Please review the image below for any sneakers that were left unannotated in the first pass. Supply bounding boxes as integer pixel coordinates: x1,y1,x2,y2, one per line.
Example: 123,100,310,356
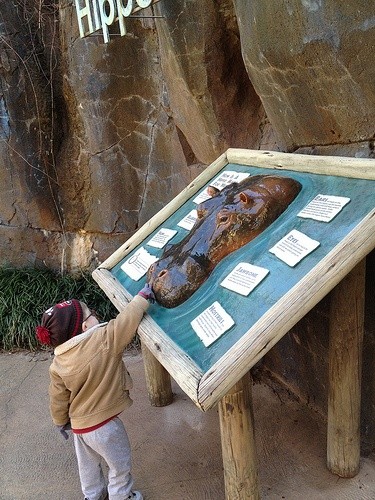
85,486,107,500
124,490,143,500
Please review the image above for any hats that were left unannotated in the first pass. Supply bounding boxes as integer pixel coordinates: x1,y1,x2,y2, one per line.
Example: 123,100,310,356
35,299,83,346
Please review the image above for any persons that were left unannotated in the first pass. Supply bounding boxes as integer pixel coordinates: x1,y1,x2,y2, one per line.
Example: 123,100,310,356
36,283,154,500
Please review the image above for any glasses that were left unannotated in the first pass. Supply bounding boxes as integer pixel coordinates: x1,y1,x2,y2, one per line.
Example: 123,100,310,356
83,309,96,321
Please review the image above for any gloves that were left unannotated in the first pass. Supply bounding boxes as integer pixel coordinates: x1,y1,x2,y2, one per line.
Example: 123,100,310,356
56,422,72,440
137,283,155,304
